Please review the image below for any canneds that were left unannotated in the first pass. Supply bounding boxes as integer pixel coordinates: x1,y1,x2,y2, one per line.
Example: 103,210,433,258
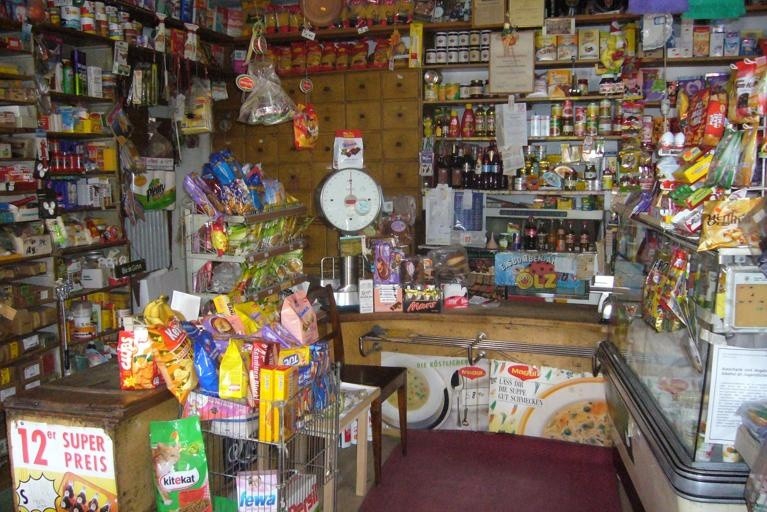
424,69,490,100
550,73,623,137
49,0,137,48
425,29,492,65
514,145,597,191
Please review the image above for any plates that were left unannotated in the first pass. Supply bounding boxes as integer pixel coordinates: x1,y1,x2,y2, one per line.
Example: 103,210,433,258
380,385,450,431
518,377,606,447
380,353,443,425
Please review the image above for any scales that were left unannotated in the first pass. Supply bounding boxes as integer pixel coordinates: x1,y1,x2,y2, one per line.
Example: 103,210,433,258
315,167,383,310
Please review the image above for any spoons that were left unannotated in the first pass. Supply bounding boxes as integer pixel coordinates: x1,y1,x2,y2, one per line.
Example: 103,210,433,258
449,369,464,428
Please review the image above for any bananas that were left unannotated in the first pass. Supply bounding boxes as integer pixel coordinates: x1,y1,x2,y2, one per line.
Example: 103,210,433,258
143,294,175,326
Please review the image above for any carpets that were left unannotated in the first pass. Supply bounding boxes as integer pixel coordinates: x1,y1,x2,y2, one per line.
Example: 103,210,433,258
359,424,625,511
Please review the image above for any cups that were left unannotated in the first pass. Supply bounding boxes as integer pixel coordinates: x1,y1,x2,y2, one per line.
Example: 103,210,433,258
514,178,527,191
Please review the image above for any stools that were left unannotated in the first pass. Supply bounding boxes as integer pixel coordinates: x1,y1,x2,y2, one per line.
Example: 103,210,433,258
253,375,381,511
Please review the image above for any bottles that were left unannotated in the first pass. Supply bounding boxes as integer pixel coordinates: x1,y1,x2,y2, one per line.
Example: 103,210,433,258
434,141,506,189
601,167,612,192
598,74,623,95
523,214,589,253
434,102,496,137
583,164,596,181
611,111,622,137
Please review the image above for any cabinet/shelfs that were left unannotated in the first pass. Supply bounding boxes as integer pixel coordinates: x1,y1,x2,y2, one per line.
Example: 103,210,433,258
213,63,421,280
2,1,236,512
422,19,641,194
591,2,766,512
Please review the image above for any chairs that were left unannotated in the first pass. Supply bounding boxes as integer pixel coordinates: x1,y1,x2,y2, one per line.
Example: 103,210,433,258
303,282,408,485
